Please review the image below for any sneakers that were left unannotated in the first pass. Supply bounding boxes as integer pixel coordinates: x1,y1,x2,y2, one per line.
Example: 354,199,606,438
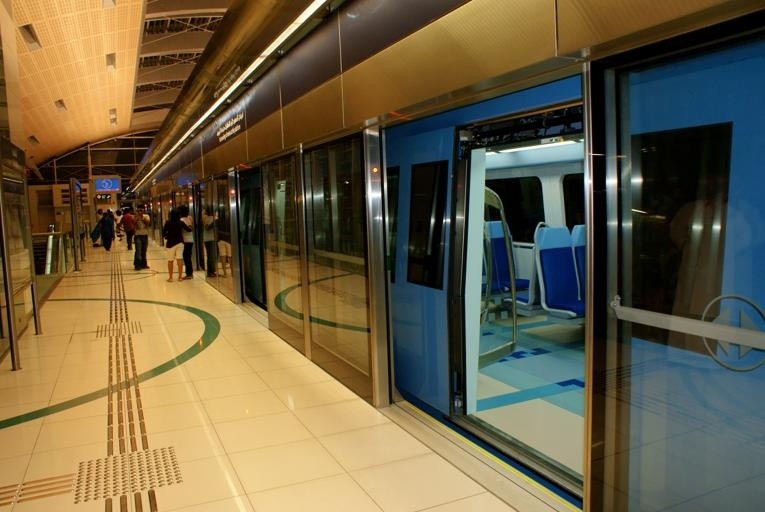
135,265,149,270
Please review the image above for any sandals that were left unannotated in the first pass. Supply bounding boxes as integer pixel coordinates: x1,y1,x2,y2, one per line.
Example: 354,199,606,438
221,274,228,277
167,276,193,282
207,272,218,277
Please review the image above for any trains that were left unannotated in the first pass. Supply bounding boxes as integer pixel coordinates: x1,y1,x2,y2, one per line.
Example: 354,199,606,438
122,0,765,512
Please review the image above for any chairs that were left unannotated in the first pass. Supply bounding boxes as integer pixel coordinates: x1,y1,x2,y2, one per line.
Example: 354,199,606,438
482,220,587,321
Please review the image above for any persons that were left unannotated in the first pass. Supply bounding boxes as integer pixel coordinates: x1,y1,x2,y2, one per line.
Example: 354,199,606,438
96,207,135,251
666,173,752,358
162,205,193,282
203,206,233,278
131,205,150,270
